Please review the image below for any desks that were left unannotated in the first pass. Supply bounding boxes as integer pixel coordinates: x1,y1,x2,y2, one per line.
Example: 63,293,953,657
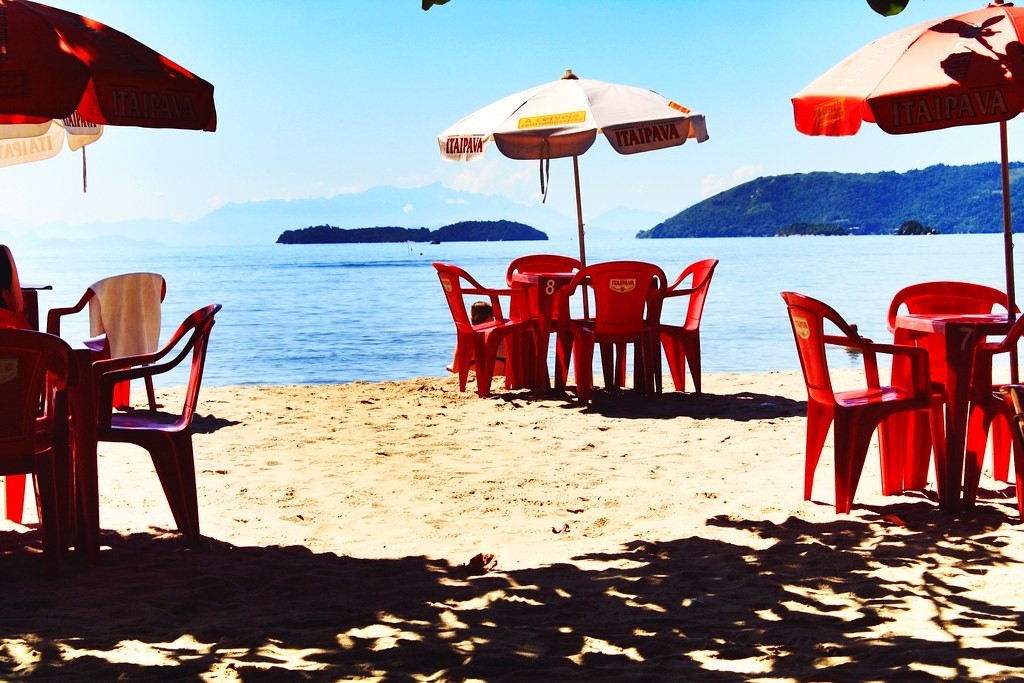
510,271,661,398
895,314,1024,516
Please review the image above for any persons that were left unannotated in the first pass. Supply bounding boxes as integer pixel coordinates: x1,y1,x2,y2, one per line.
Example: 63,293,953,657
446,301,505,376
0,244,31,330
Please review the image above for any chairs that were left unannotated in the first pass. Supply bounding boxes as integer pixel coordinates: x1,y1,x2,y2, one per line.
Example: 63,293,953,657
431,255,718,408
781,280,1023,525
0,244,222,565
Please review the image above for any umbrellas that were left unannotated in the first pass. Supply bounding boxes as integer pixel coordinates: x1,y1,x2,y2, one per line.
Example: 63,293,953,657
437,68,709,320
791,0,1024,384
0,0,217,170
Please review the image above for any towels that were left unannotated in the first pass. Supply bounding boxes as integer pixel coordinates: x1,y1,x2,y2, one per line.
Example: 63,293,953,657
87,272,163,360
